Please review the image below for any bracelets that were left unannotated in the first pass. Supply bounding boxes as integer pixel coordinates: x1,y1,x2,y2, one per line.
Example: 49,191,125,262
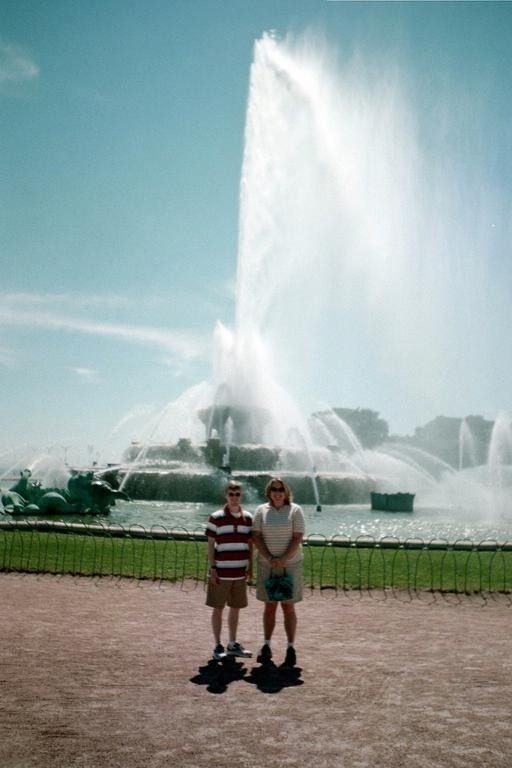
268,556,273,561
210,565,217,569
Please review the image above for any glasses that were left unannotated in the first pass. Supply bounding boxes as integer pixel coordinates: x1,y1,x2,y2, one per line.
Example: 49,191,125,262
228,492,241,496
270,486,284,491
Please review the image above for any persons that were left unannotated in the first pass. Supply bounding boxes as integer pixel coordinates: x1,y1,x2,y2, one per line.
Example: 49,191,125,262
205,481,253,661
253,478,305,664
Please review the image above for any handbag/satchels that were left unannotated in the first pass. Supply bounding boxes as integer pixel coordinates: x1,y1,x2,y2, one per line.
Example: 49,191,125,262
264,574,293,602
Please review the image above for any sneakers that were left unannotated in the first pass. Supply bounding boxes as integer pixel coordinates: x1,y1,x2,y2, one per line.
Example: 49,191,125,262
286,645,297,660
257,643,271,661
212,642,253,661
